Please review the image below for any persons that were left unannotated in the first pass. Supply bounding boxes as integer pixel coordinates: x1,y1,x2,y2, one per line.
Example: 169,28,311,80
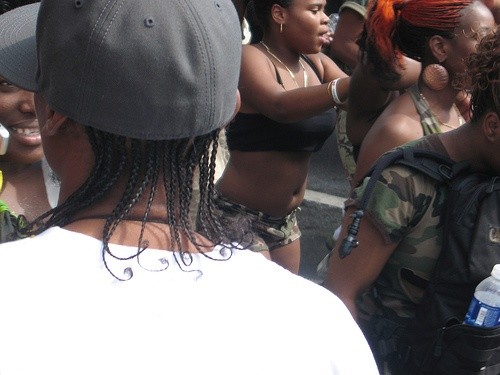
0,0,500,375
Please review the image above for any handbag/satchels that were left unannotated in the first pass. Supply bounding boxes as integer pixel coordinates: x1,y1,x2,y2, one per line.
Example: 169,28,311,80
344,61,400,145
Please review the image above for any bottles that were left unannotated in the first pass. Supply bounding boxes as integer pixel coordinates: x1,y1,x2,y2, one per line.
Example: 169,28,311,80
327,13,339,33
460,263,500,327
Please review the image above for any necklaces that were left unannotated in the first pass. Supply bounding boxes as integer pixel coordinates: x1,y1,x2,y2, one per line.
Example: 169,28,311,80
59,214,191,229
420,92,462,129
259,41,307,89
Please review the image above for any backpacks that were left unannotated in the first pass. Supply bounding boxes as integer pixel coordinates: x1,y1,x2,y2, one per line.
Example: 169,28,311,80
339,148,500,375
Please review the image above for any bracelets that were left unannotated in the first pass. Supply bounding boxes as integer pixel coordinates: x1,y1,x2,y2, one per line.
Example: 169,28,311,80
332,78,344,105
327,81,333,98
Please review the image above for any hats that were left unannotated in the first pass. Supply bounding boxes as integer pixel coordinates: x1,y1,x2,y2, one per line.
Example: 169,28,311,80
0,0,241,141
